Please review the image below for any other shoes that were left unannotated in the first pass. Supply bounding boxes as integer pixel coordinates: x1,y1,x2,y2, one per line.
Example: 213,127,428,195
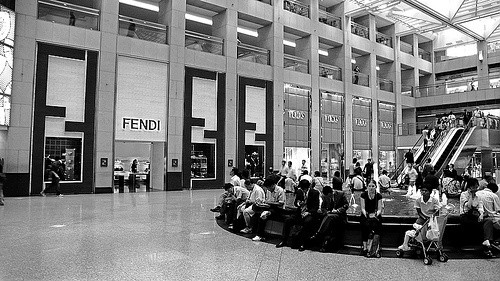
57,193,63,197
252,235,265,241
309,232,320,240
40,191,47,197
215,215,225,220
276,240,288,248
260,211,271,220
240,227,252,234
210,206,221,212
299,244,305,250
483,240,491,247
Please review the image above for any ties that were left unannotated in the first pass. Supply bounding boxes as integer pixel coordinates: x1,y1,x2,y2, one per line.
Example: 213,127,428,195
305,192,306,196
331,197,334,208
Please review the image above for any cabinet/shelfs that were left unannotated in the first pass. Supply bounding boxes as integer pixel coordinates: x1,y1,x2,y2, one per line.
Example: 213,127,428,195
190,150,207,178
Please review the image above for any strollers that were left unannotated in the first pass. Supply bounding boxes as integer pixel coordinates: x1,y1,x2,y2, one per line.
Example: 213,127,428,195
395,204,450,266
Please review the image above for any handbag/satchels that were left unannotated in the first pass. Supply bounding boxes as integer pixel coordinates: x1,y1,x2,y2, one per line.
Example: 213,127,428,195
426,215,440,241
468,209,489,219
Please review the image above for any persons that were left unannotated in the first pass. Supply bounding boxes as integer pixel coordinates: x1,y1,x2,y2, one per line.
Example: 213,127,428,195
376,33,389,46
68,12,75,26
126,18,136,37
40,158,66,198
210,148,500,258
283,0,307,16
353,66,360,85
421,106,500,152
131,159,138,173
351,21,369,39
319,12,338,27
464,75,500,92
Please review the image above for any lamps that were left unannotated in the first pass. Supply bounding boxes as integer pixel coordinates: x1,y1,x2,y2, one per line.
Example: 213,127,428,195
478,50,484,64
